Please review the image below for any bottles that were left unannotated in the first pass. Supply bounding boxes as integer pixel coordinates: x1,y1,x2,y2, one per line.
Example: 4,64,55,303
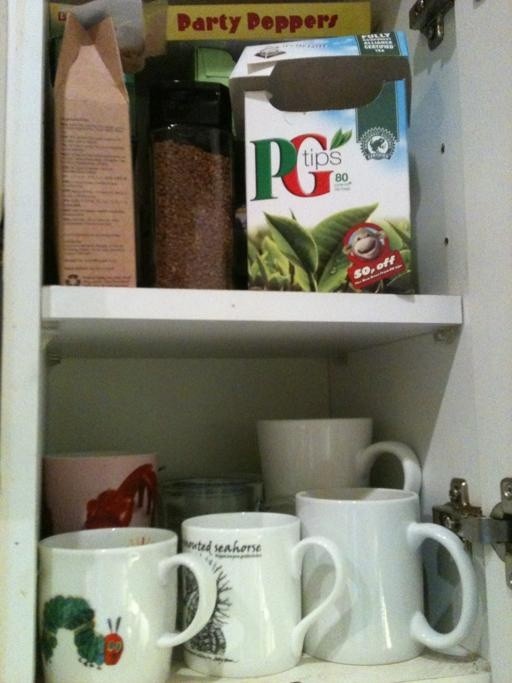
147,74,238,287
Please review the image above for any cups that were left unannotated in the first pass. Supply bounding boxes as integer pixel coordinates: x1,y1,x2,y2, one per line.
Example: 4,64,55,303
157,475,264,526
249,415,423,509
182,508,344,680
297,483,477,667
42,449,158,531
37,526,220,681
